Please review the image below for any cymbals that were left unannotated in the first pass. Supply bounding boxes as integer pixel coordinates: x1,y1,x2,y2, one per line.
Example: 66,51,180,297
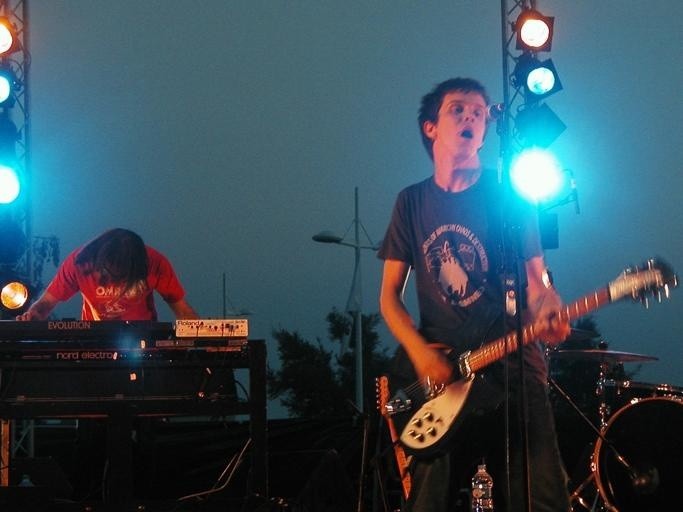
547,348,660,361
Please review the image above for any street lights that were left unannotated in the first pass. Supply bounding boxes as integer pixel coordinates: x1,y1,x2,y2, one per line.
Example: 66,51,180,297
312,187,378,407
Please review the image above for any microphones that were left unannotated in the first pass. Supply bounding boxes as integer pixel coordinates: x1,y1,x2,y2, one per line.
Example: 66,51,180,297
484,101,505,122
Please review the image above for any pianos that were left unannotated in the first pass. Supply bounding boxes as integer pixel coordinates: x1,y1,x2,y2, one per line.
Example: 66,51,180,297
0,320,251,400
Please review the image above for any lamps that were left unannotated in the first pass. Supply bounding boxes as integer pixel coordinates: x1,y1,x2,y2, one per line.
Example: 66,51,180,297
514,104,567,151
0,15,23,206
515,53,542,88
516,57,564,101
515,9,555,50
0,278,31,314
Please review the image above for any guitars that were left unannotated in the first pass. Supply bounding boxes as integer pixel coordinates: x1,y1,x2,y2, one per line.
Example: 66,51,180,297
373,257,677,464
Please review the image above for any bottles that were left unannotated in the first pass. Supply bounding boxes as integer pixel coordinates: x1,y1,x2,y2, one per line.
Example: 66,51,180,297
471,464,494,512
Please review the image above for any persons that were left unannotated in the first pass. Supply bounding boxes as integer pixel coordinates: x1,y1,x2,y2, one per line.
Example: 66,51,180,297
375,76,573,512
15,227,205,501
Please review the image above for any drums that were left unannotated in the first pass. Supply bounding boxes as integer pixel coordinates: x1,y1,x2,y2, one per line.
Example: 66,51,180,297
590,398,683,511
595,380,683,415
